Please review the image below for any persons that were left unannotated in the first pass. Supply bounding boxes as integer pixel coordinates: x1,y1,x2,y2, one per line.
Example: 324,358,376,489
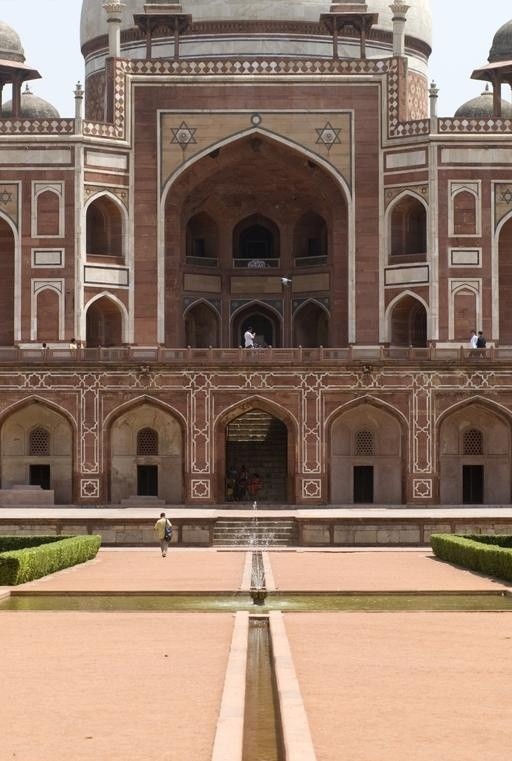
243,326,256,349
154,512,173,559
476,331,488,358
470,329,478,349
227,464,261,500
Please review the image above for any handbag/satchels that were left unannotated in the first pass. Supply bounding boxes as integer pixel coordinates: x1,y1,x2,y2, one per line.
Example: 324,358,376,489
165,518,172,541
